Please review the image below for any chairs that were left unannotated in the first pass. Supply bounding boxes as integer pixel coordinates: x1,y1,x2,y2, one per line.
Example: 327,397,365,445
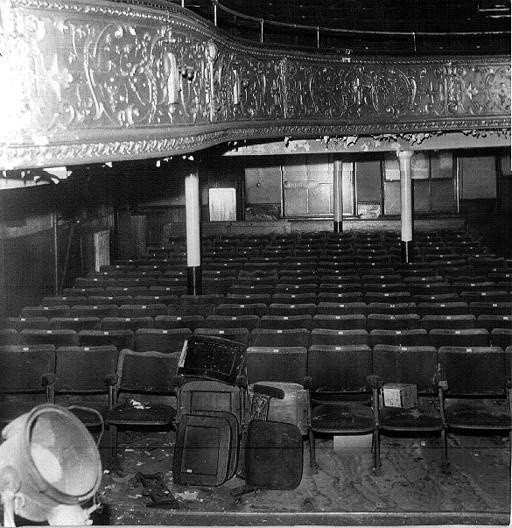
106,349,181,457
245,345,314,472
307,345,379,474
438,345,507,475
1,228,510,353
505,345,512,412
1,344,55,429
372,344,447,473
42,345,118,454
